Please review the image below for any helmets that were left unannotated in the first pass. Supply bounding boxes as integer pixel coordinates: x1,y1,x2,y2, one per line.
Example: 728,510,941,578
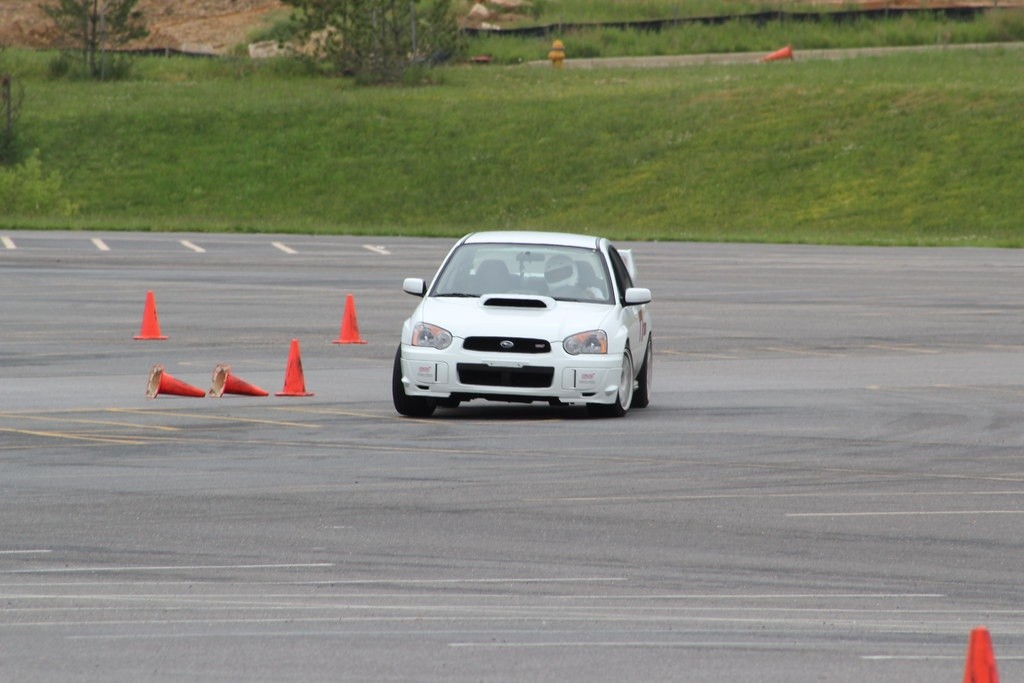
543,254,577,292
476,259,510,295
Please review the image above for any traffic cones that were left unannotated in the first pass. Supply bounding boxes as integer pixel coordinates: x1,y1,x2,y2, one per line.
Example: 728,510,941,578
132,290,169,341
145,364,207,400
760,43,794,62
274,339,315,397
207,361,269,398
330,293,370,344
962,627,999,683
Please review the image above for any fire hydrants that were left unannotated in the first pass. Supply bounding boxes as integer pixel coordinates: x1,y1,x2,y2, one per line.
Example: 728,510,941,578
548,40,565,70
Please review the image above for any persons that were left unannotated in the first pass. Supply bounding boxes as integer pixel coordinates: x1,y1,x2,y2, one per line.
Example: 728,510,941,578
544,254,605,300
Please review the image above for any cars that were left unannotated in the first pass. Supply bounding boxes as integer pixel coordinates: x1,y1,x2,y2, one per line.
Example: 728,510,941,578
391,230,653,418
